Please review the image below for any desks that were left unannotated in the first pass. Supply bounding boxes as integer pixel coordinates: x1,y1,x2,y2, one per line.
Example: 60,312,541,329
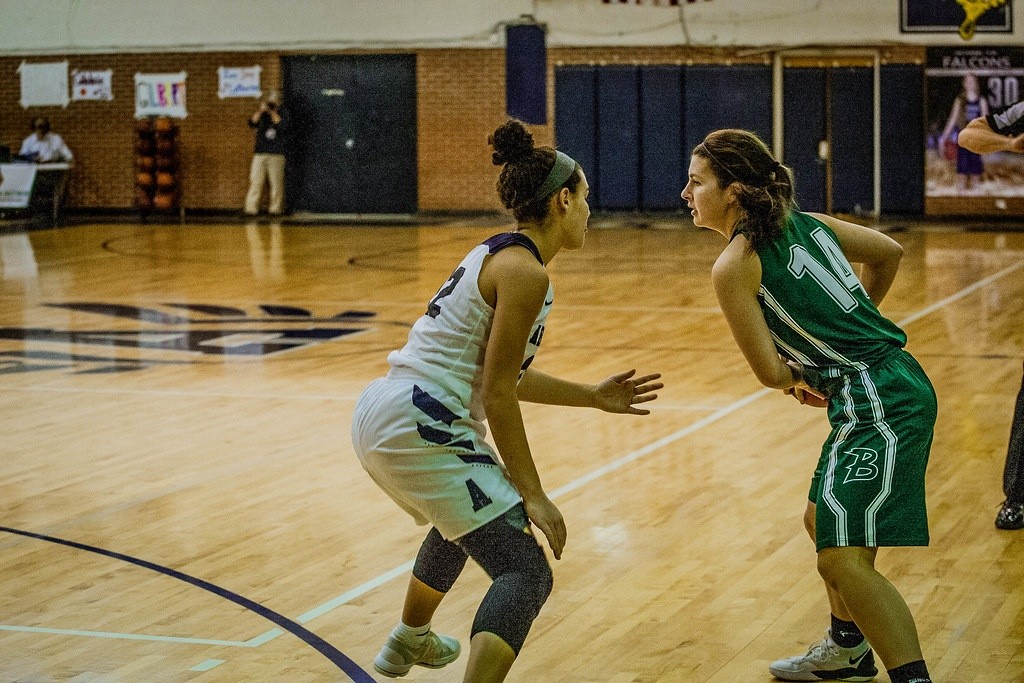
0,160,75,232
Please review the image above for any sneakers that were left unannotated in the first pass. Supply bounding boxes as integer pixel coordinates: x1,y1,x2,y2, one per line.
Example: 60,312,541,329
769,626,880,682
371,626,460,678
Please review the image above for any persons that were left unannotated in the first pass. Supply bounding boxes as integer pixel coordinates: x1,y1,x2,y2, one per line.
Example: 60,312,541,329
959,101,1024,529
239,90,288,221
350,120,665,682
683,128,937,683
19,119,77,219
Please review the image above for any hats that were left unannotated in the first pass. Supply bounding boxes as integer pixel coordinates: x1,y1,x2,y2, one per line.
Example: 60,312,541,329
267,88,287,103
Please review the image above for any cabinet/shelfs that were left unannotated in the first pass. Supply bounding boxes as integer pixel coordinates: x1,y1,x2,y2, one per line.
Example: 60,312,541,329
130,123,187,225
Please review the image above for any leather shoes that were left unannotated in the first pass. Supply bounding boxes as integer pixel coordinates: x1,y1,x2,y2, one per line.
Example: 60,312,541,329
995,495,1024,530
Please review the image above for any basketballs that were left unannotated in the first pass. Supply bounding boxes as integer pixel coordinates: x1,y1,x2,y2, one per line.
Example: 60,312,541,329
779,354,828,409
133,114,180,211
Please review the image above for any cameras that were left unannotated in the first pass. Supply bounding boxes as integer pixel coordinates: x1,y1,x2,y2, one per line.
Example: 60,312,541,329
268,101,277,109
